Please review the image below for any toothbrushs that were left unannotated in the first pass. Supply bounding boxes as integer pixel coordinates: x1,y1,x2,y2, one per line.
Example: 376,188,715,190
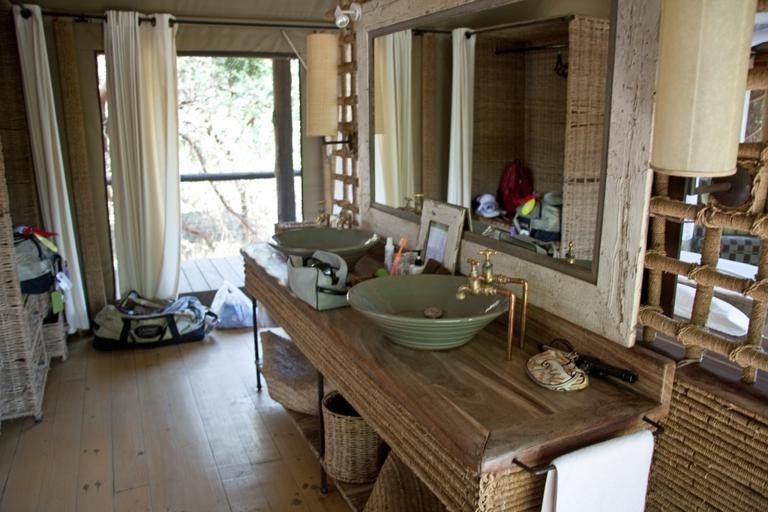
390,238,409,274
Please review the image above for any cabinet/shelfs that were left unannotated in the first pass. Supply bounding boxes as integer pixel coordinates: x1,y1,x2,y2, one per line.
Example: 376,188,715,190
239,291,675,512
0,43,67,428
418,15,612,259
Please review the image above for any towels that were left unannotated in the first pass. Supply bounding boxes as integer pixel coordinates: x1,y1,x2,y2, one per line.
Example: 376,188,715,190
541,429,656,511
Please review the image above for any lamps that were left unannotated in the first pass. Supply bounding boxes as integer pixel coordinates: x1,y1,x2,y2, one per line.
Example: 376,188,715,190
304,35,357,157
331,2,364,29
651,1,753,208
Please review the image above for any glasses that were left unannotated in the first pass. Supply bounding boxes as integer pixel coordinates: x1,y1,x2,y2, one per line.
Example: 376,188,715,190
304,257,334,277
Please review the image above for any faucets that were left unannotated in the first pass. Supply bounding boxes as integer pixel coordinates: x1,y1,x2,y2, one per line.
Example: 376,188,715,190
315,199,331,226
478,249,529,351
457,256,517,362
333,196,360,230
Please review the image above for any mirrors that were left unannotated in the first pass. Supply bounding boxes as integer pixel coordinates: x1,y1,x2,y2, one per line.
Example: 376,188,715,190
369,2,620,285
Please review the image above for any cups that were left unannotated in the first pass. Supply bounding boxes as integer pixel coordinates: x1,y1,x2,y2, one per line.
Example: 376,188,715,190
392,253,412,276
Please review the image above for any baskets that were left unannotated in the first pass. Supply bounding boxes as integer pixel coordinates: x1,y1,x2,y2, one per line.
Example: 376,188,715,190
323,388,385,488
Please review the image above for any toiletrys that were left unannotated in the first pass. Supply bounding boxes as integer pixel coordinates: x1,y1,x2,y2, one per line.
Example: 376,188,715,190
384,236,395,271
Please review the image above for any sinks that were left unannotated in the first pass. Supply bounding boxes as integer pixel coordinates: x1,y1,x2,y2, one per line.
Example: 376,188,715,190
345,272,512,352
273,224,383,272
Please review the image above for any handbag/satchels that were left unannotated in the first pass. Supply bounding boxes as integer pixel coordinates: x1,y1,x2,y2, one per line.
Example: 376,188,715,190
525,349,590,394
90,291,217,353
286,248,354,314
500,162,536,217
513,192,562,242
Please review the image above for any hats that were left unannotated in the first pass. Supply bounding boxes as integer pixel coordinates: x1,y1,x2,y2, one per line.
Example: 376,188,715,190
473,193,508,219
